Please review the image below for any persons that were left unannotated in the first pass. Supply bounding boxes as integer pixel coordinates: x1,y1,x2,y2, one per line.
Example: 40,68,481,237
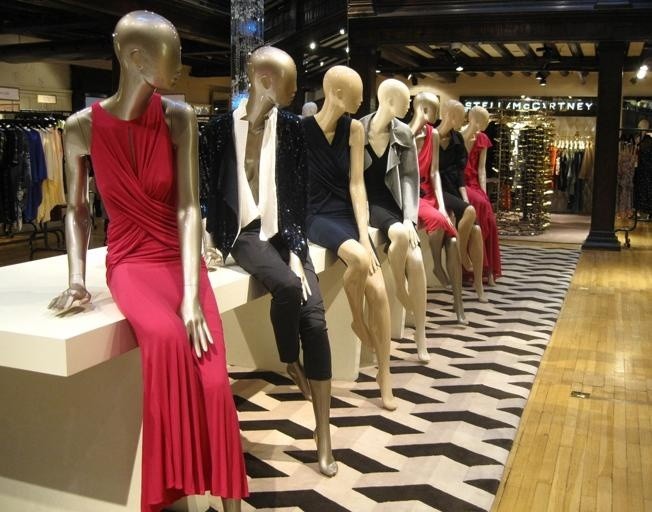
345,76,431,365
189,43,338,478
453,104,503,285
428,97,491,305
45,9,251,510
395,90,473,327
291,63,399,412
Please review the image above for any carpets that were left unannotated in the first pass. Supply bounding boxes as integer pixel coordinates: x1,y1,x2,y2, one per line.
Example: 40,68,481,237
203,241,582,512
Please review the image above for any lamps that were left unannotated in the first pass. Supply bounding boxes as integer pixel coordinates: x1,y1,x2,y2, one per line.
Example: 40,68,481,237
402,73,418,85
535,71,547,86
